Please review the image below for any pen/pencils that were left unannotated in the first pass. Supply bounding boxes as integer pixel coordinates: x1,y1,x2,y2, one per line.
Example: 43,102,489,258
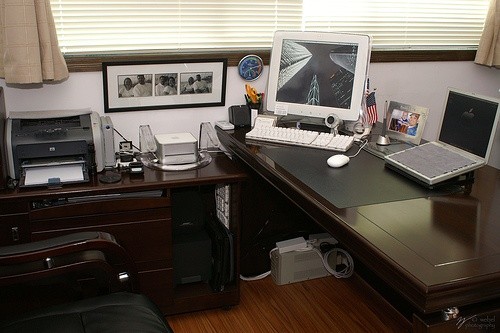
244,92,264,103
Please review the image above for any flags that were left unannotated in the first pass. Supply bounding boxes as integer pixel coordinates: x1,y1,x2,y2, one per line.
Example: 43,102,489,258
365,91,378,125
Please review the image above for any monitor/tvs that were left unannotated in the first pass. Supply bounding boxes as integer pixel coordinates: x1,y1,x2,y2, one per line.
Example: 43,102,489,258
266,31,371,122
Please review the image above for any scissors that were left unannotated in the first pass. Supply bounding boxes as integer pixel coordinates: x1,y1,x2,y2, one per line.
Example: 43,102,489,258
245,84,257,103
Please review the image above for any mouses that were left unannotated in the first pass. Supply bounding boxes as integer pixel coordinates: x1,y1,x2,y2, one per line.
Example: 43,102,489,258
326,154,350,167
377,134,390,145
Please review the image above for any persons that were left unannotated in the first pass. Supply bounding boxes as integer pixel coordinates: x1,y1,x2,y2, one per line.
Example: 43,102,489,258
134,75,152,97
155,76,167,95
193,74,209,94
181,77,194,94
119,78,134,98
161,77,177,95
393,111,421,136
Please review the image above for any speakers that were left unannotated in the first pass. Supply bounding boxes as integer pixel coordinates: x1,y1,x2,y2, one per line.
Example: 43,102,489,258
172,191,207,235
228,105,250,125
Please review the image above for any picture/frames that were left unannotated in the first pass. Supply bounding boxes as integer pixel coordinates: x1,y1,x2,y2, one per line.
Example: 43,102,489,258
381,101,429,144
102,58,227,113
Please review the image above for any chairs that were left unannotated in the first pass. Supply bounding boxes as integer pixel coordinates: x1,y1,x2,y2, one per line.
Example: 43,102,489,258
0,232,173,333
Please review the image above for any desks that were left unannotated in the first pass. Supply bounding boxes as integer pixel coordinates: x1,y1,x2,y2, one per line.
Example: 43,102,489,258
217,122,500,333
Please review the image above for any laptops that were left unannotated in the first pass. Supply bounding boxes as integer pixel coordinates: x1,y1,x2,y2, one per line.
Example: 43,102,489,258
383,86,500,185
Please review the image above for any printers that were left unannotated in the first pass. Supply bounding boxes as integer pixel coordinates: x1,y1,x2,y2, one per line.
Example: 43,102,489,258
4,109,115,188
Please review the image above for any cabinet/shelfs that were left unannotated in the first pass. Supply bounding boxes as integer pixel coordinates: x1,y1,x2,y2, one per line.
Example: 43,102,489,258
0,148,246,317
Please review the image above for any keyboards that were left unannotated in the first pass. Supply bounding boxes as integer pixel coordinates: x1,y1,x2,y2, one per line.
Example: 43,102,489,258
245,125,354,152
